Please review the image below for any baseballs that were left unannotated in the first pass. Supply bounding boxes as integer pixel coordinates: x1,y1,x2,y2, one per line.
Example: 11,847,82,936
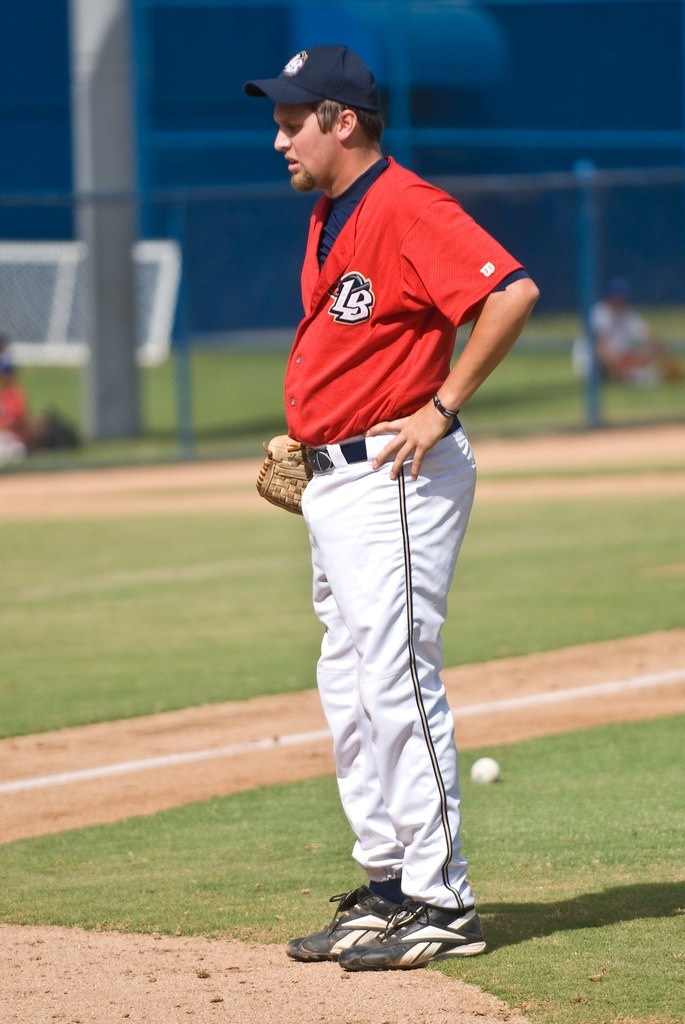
472,757,503,788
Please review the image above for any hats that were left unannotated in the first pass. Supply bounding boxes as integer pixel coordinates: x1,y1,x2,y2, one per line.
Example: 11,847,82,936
243,44,380,111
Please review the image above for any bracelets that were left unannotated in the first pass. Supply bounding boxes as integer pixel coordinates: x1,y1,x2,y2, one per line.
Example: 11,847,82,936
433,393,460,418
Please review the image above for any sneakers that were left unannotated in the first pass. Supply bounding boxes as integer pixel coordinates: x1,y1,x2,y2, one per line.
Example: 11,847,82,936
338,900,486,971
286,886,401,962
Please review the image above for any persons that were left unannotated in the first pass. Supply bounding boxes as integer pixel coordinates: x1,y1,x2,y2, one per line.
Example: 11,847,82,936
0,343,87,467
572,274,685,383
244,47,541,972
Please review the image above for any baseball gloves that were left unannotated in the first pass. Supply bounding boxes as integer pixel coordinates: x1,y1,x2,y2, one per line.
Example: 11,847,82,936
255,434,314,516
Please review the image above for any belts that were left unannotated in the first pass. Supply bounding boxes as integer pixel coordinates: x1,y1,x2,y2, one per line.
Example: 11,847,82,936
306,415,462,472
